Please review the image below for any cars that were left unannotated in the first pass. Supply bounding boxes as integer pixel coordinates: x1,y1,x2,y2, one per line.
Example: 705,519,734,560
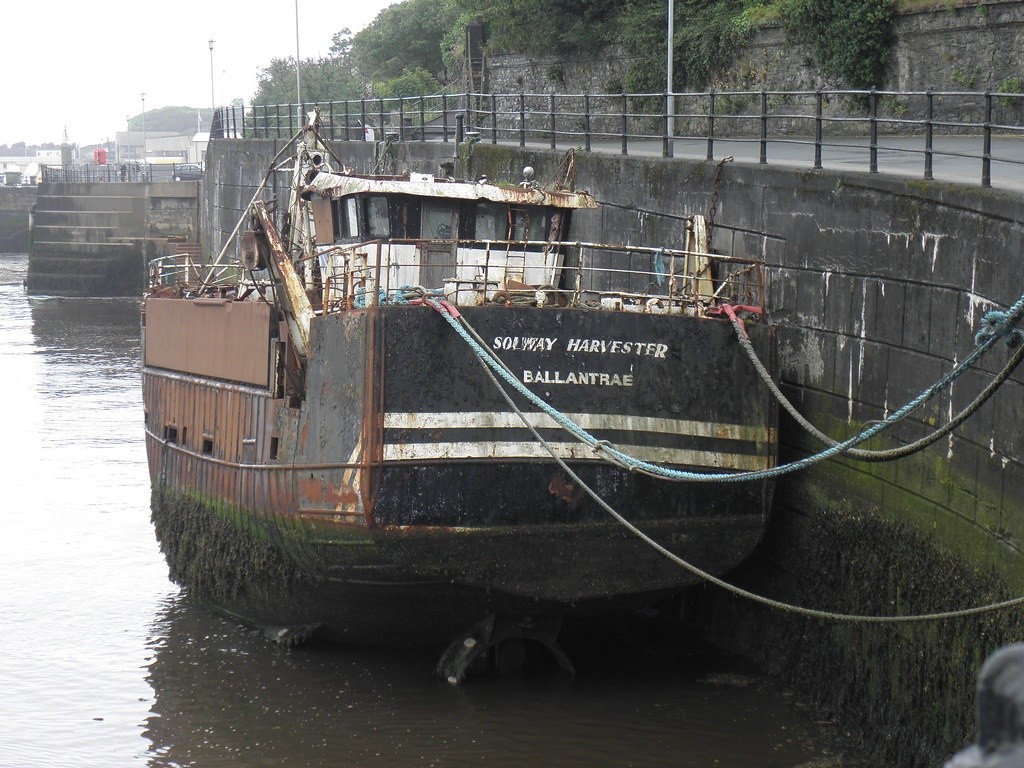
172,163,205,181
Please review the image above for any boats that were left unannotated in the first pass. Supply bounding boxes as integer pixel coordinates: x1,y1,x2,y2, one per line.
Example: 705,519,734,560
140,103,779,686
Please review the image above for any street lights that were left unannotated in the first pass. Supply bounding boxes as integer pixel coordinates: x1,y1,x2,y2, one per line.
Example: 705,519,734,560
209,37,216,113
